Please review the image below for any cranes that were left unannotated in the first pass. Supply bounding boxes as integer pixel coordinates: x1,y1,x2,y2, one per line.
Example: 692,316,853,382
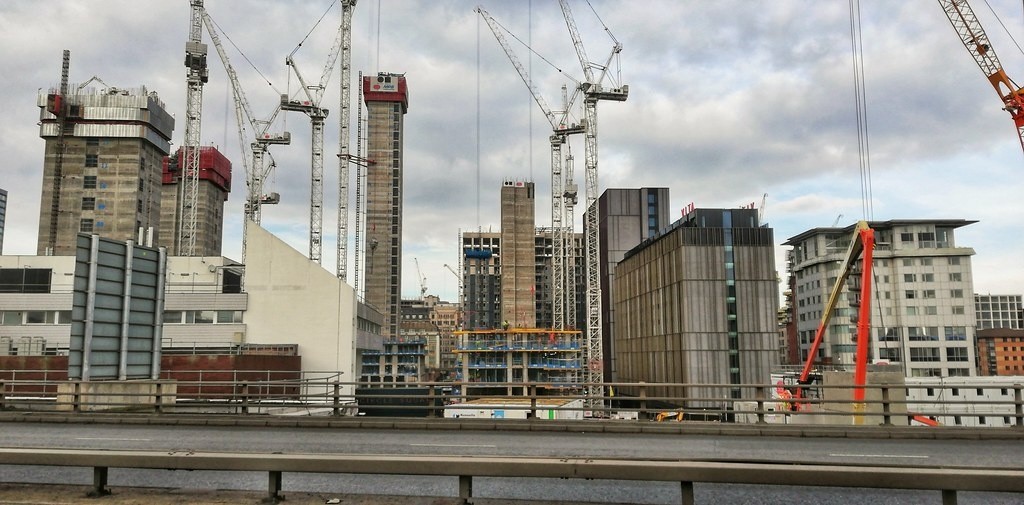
468,0,630,408
171,0,351,285
